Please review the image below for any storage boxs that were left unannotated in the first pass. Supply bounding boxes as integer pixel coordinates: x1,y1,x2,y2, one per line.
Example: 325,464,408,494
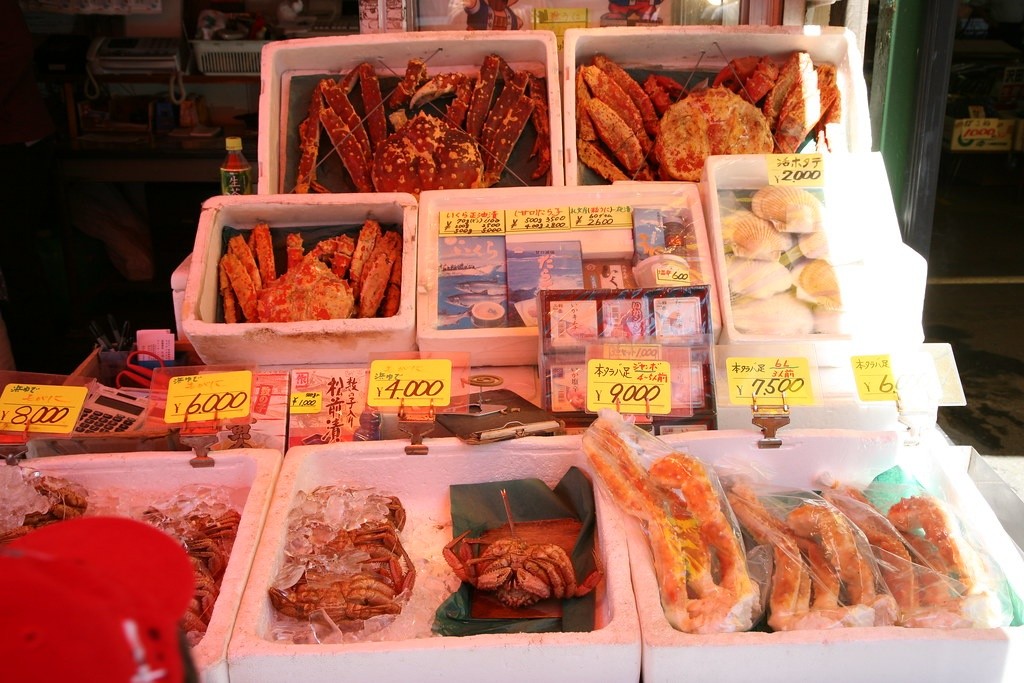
625,428,1024,683
181,191,417,362
0,447,283,683
124,0,183,39
228,434,642,683
699,150,928,345
418,183,723,366
562,23,873,183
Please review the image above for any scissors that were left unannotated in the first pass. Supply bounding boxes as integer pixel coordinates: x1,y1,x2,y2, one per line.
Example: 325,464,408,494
116,351,172,391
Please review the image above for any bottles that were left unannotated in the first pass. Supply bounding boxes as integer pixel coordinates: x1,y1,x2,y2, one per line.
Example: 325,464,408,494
219,136,252,196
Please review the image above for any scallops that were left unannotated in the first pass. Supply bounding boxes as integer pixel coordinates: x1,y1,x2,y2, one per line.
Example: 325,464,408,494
720,185,843,334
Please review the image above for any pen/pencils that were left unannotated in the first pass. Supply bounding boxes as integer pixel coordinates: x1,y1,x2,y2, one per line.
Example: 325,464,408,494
91,314,129,352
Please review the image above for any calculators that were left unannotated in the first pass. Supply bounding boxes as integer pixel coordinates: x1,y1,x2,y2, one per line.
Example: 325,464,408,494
72,382,158,433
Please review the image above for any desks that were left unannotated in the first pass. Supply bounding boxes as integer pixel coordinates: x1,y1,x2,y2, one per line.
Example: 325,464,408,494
36,141,257,333
258,29,564,193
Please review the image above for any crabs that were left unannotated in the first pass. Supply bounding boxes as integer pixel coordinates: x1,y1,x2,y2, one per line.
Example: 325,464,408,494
442,529,605,610
582,416,973,634
141,485,416,649
218,52,841,324
